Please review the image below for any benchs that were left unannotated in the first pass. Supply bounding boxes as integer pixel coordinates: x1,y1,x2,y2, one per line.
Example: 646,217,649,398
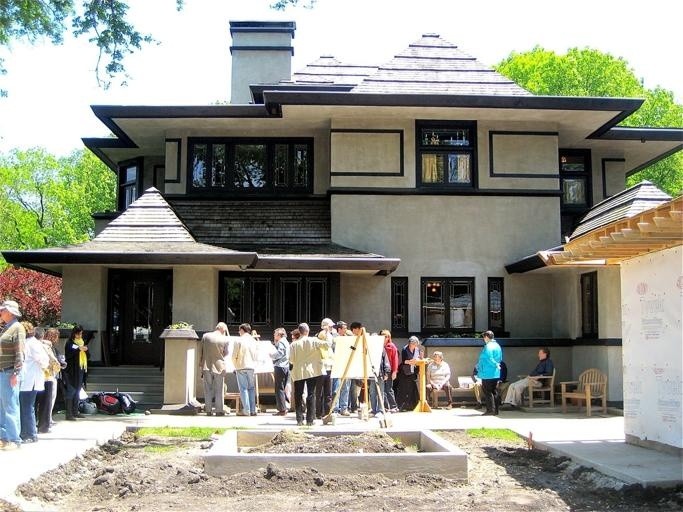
428,383,499,408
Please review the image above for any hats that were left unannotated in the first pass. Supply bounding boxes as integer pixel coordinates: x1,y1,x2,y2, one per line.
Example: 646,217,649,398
0,301,22,317
320,318,336,329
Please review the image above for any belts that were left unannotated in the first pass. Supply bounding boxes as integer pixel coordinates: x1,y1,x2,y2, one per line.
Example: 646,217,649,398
0,366,14,372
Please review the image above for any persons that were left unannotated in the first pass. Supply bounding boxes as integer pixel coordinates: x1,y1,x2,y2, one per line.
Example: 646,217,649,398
500,347,554,409
198,317,453,426
15,321,49,442
45,327,62,424
0,300,26,450
33,326,60,434
477,330,502,416
471,360,511,410
59,326,91,421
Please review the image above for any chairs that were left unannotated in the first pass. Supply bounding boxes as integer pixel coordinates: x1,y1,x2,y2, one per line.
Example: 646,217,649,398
559,367,608,416
516,367,556,408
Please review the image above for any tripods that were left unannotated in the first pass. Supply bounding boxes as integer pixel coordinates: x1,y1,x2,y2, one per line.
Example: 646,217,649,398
322,328,394,428
